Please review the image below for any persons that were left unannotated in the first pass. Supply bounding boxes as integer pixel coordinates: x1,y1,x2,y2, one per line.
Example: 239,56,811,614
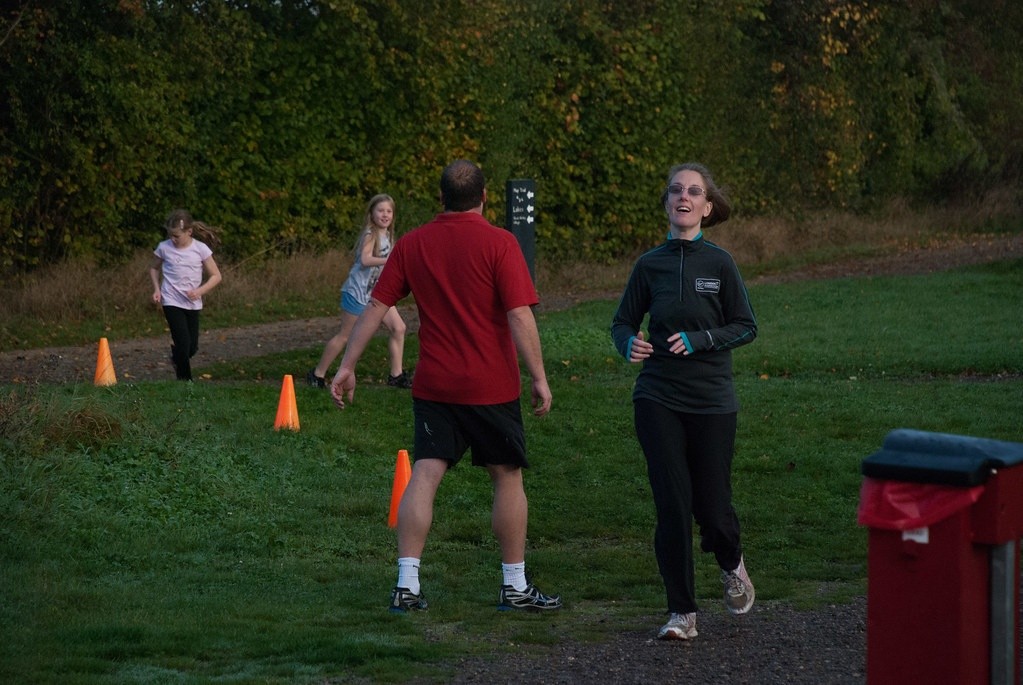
330,161,572,609
149,210,226,381
611,161,763,640
307,192,416,389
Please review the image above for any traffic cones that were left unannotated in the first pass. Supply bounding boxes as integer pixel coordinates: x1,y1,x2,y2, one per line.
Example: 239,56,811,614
273,373,300,431
93,337,118,388
385,449,413,528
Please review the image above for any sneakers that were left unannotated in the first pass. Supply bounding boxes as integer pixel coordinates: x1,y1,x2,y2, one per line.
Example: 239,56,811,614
389,587,429,613
306,369,325,388
497,581,564,611
720,553,756,614
657,612,698,639
386,370,413,389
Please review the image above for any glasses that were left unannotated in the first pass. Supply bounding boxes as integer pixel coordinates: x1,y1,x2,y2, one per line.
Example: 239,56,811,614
668,185,707,197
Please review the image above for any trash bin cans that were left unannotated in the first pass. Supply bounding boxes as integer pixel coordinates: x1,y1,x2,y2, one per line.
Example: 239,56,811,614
854,427,1021,684
